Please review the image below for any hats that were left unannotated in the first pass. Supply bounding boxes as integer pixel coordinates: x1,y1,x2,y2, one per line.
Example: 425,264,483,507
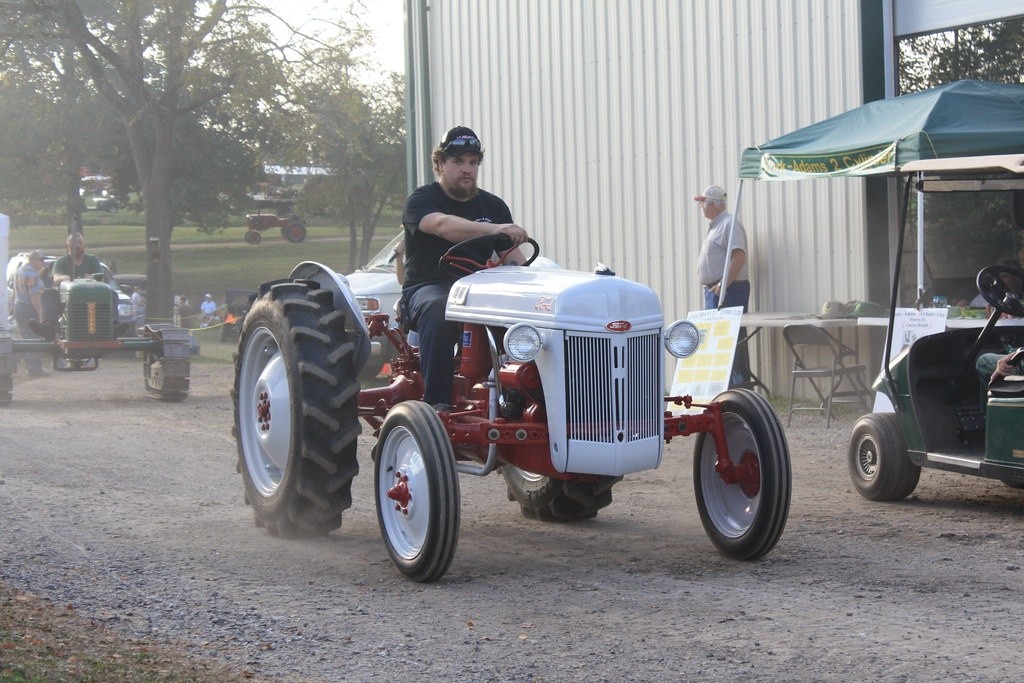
28,250,49,261
439,126,482,158
815,300,846,319
848,302,882,316
694,185,727,202
206,293,212,298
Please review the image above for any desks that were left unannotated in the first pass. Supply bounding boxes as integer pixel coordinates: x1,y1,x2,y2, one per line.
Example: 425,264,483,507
739,312,1024,418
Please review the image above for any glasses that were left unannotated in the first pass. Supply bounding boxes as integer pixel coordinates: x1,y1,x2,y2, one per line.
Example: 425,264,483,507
444,138,481,150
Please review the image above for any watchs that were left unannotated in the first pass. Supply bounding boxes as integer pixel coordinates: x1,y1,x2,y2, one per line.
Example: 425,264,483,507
718,283,722,287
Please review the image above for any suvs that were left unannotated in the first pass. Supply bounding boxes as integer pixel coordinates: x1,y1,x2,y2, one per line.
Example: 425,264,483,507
6,252,134,340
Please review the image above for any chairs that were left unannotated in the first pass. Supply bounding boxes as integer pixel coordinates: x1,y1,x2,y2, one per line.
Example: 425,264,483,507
989,352,1024,404
388,240,420,334
782,324,875,428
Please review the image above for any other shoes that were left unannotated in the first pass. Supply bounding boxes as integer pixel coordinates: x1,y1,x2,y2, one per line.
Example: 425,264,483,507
29,318,54,342
113,320,129,337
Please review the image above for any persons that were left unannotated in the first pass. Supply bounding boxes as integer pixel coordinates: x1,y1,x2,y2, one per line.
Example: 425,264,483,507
131,285,144,326
693,185,753,389
995,257,1024,300
29,233,105,341
199,293,216,328
399,125,528,413
976,349,1024,420
178,295,192,327
7,249,50,376
109,259,116,275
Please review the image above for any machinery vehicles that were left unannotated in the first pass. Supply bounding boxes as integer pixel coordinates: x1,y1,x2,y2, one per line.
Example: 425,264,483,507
233,235,793,587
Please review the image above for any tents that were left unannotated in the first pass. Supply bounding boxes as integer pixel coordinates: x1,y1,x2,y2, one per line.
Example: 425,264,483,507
717,78,1024,308
